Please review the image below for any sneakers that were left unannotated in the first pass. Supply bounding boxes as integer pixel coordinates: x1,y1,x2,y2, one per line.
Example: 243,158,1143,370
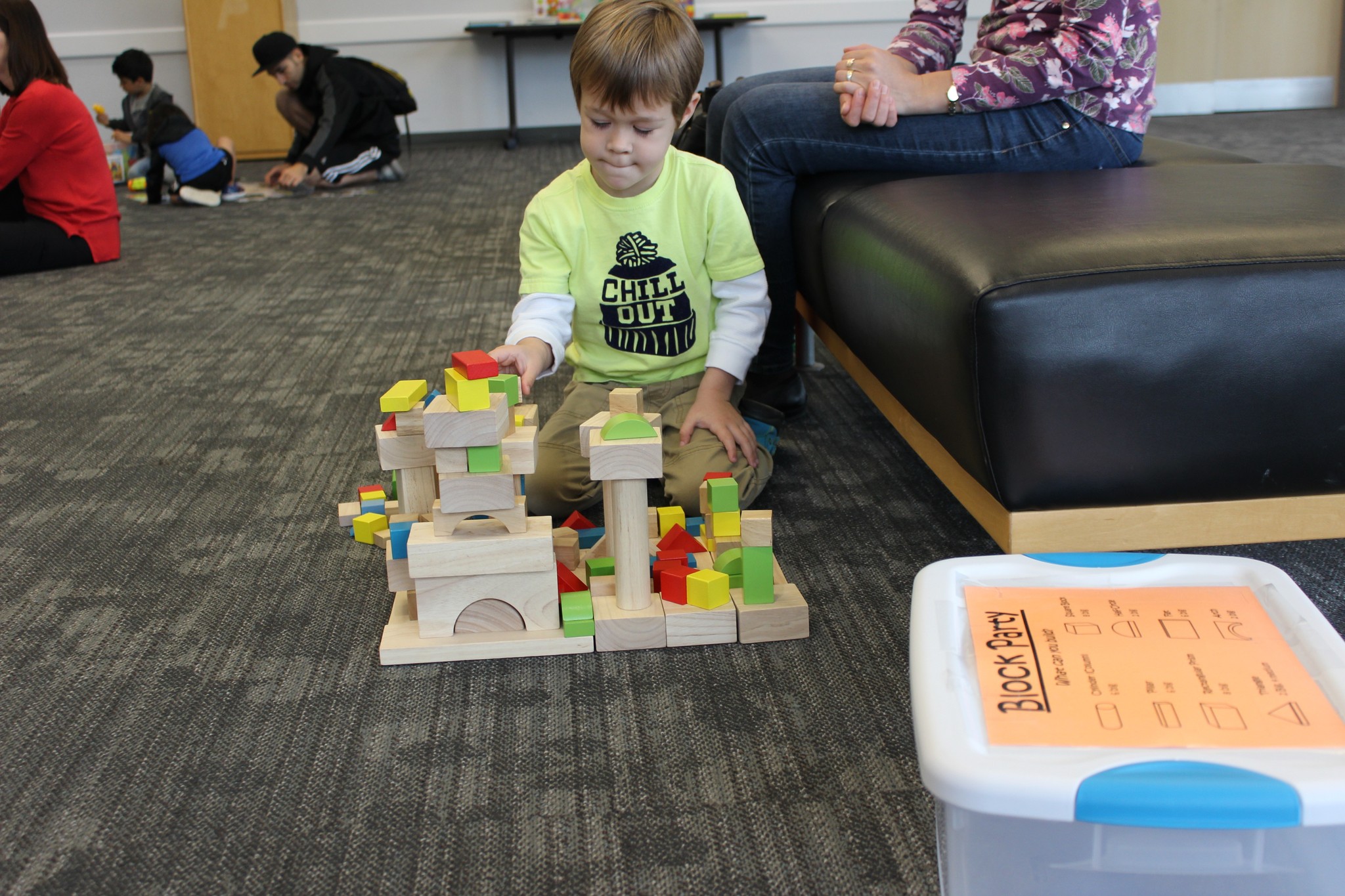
222,181,247,203
741,399,784,456
377,159,404,181
127,176,147,191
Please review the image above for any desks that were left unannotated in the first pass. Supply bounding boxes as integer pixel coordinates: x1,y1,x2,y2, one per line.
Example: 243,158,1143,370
464,12,766,153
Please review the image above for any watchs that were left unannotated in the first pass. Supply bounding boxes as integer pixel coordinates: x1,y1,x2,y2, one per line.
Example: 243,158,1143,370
946,81,959,116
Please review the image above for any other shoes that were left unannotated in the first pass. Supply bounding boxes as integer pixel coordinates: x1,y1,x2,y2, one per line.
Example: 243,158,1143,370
741,371,809,425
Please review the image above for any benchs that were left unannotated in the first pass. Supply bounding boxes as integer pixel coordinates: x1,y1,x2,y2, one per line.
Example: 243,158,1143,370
788,130,1344,557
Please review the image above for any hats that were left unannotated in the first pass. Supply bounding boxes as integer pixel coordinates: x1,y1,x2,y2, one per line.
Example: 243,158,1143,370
252,33,297,77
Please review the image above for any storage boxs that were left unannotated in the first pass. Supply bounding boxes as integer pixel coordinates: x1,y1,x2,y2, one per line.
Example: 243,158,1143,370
906,548,1345,896
103,140,140,186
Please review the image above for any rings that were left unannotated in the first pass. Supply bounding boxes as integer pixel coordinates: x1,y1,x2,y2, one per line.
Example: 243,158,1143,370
846,58,855,70
847,70,854,82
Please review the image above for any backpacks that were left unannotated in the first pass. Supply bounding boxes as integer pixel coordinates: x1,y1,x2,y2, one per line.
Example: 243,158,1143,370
330,56,417,115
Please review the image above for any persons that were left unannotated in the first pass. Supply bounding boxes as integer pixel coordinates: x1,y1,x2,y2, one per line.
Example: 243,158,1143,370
0,0,122,277
250,30,418,191
97,48,177,181
134,100,247,207
704,0,1159,413
486,1,784,531
